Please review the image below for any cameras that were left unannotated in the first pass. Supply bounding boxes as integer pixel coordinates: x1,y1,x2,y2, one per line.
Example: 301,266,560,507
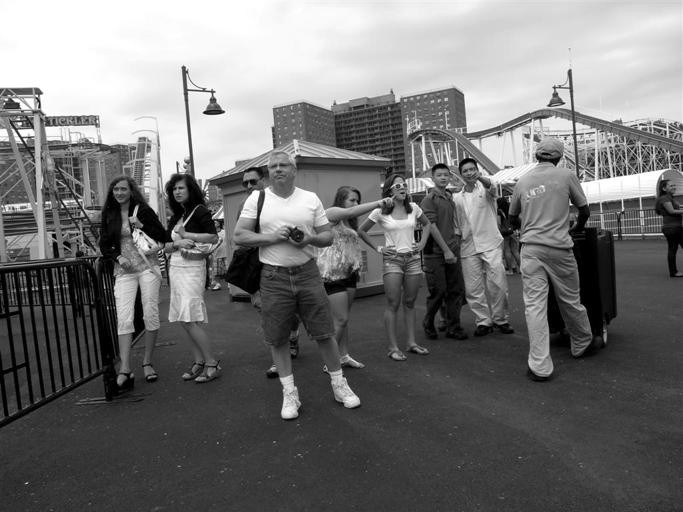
287,226,306,242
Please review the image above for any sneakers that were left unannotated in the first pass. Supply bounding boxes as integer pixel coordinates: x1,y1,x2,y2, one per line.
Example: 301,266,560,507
423,321,438,338
341,353,366,369
496,322,513,334
442,327,466,339
671,272,682,277
267,364,279,378
281,388,301,419
506,270,513,275
289,337,299,359
527,366,555,382
474,324,493,336
517,269,522,274
574,336,604,358
213,283,221,291
331,376,360,408
323,365,329,374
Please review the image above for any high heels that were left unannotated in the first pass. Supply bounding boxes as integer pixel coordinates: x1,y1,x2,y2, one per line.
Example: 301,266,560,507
112,372,134,393
142,365,158,383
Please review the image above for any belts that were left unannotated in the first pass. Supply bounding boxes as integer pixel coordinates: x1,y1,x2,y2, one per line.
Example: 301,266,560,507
395,251,418,258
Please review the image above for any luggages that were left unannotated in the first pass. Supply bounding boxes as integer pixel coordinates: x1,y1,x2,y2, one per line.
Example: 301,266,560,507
547,226,617,346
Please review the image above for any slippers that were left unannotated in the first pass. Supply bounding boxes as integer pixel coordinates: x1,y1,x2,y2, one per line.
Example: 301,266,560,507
388,348,406,360
409,344,429,355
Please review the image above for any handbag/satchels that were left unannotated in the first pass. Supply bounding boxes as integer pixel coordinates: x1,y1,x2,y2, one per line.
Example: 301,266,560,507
499,221,513,238
132,229,164,257
179,236,223,260
223,246,263,295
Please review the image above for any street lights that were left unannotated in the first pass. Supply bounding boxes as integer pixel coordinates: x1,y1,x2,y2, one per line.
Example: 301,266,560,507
547,66,583,183
179,66,225,178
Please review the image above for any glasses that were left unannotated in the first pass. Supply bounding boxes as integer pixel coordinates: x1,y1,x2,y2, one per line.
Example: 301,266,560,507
267,161,293,169
243,177,262,187
391,183,407,189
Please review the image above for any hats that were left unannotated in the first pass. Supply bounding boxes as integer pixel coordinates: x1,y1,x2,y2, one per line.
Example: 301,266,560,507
536,138,564,158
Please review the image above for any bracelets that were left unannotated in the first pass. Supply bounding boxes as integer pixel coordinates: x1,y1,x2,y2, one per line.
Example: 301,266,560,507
377,245,383,255
378,199,382,207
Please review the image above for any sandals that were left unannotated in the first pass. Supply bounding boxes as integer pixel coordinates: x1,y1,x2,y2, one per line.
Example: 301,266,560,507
181,361,204,380
195,360,222,383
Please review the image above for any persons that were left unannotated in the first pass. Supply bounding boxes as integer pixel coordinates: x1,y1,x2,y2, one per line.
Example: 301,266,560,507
235,150,362,421
156,217,225,291
99,176,166,387
319,185,396,376
236,166,300,377
70,252,94,294
509,136,602,381
164,174,222,384
421,137,521,341
358,175,432,362
655,180,683,278
